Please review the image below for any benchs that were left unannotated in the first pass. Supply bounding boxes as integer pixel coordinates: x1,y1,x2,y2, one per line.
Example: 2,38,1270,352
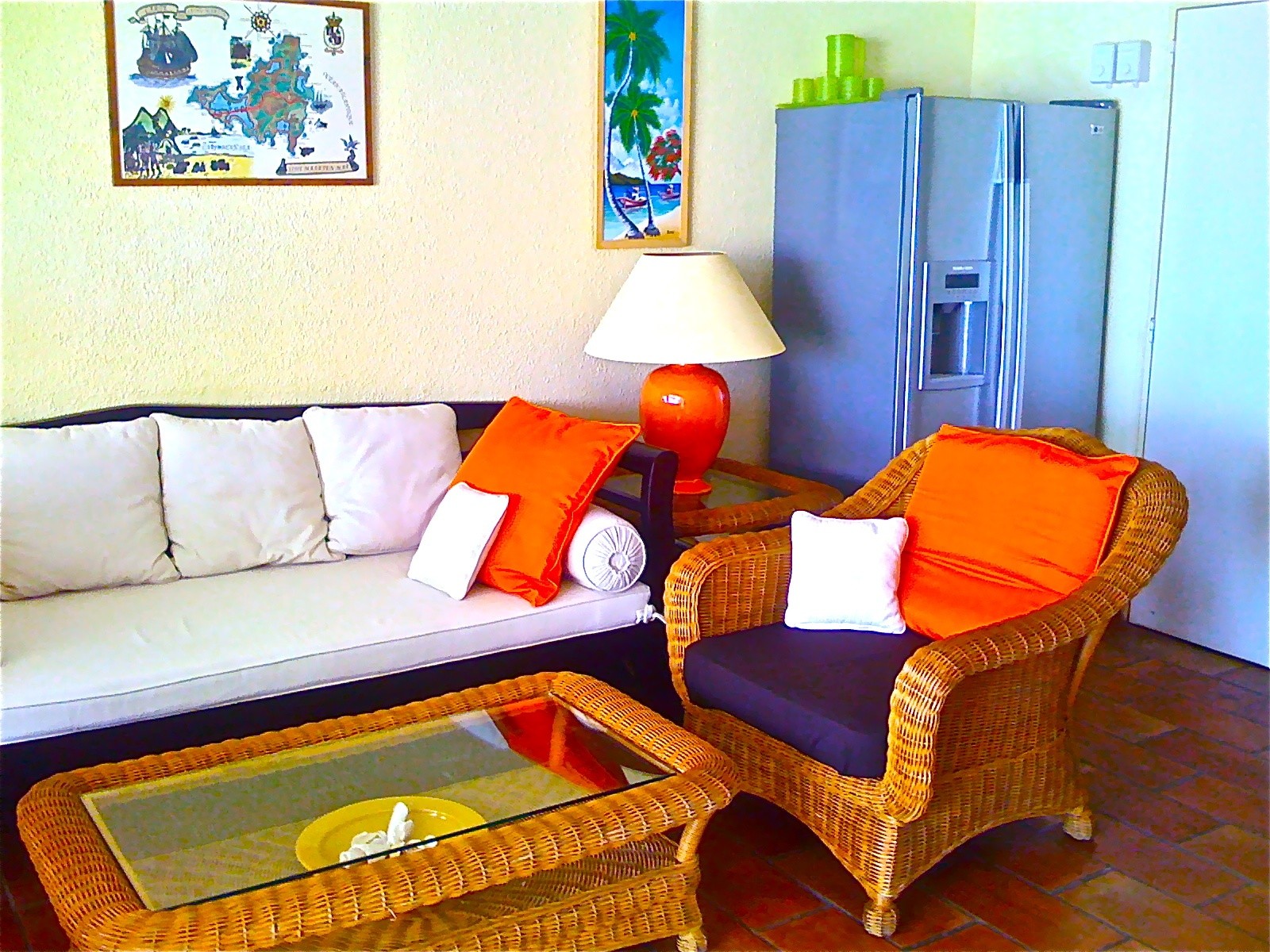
0,401,680,813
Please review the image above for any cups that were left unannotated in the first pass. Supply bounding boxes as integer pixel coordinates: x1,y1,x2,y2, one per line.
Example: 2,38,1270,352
862,77,884,102
816,76,838,103
839,76,863,102
792,78,816,104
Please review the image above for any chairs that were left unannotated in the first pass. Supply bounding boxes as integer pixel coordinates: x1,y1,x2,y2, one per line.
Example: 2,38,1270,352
662,427,1190,939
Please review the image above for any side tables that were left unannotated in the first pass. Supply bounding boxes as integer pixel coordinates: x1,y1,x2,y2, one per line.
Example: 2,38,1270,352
593,458,845,542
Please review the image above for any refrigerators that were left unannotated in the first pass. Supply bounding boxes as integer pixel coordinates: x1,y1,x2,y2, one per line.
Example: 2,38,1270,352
770,88,1118,497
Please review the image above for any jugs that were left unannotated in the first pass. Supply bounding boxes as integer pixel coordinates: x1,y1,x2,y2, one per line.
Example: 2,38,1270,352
826,34,866,101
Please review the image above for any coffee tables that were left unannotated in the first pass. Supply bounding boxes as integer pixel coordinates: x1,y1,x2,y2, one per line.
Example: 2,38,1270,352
18,670,743,952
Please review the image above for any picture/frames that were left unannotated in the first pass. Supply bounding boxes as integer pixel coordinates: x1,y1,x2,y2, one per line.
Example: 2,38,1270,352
103,0,375,188
594,0,693,249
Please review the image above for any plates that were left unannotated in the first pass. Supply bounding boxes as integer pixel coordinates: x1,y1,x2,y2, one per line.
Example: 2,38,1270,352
296,796,488,877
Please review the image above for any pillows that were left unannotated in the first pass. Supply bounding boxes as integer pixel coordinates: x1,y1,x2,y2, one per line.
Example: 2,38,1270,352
149,412,346,578
785,508,907,635
0,418,182,602
897,423,1139,642
448,394,643,609
407,481,511,600
562,504,647,594
303,402,464,556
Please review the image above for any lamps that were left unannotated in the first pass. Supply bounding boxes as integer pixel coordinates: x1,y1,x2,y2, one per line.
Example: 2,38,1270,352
584,248,785,498
